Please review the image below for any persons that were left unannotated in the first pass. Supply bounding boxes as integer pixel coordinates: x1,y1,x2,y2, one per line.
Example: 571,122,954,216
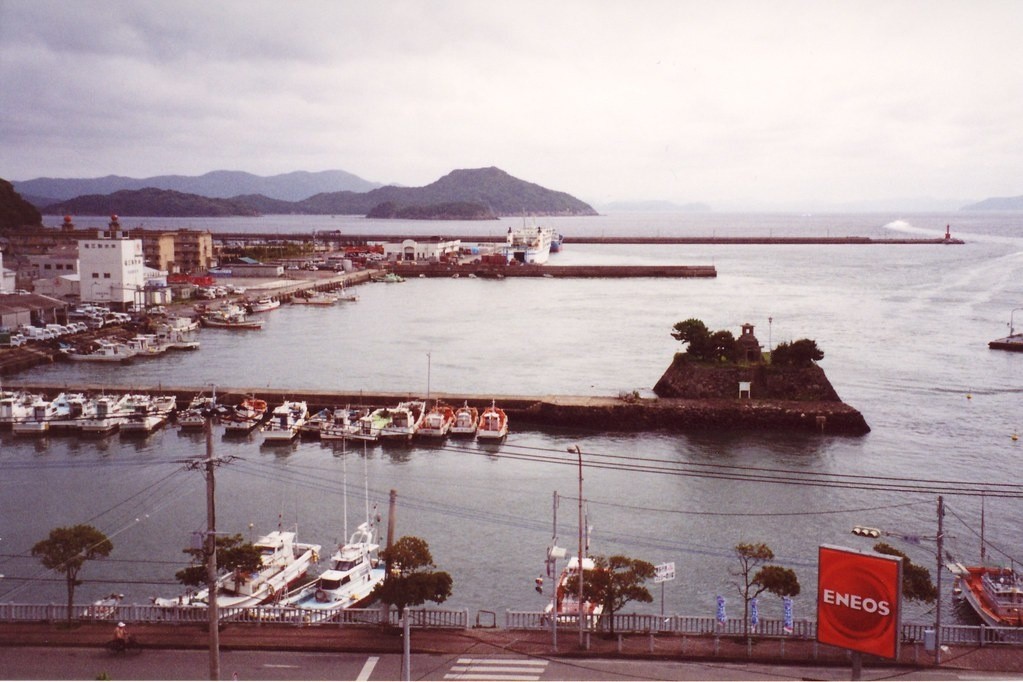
114,621,129,659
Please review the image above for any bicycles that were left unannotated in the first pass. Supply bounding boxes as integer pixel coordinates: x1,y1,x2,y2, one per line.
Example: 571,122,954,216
104,633,142,658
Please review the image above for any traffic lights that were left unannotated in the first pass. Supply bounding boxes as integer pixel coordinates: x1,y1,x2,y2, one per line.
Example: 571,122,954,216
943,562,971,580
951,587,963,604
534,577,544,594
852,525,882,539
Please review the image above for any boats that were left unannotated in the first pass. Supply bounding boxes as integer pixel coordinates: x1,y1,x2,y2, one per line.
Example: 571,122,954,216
494,205,551,265
246,433,404,627
370,270,405,282
0,282,281,363
544,526,606,629
148,485,323,624
76,592,126,620
549,227,565,253
290,277,337,305
326,281,360,302
960,491,1023,642
0,382,510,445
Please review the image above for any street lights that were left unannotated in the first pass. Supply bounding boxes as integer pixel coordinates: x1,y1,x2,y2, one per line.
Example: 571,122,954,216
425,351,432,410
566,443,585,648
767,315,773,353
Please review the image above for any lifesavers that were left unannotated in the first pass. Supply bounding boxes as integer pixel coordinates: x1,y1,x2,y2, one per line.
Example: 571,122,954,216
267,585,276,599
314,590,327,602
